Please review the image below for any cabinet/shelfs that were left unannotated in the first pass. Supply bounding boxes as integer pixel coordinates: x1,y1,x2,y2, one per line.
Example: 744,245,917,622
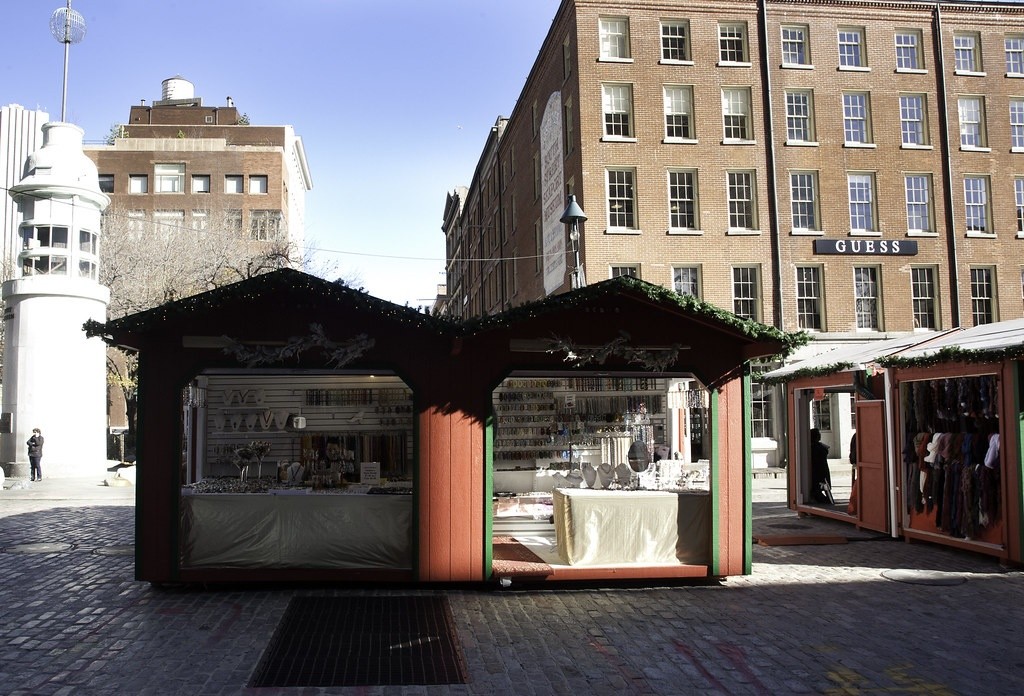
210,406,288,434
493,496,554,530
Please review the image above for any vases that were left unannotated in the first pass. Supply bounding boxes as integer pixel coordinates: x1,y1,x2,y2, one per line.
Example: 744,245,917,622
256,456,265,479
241,459,249,484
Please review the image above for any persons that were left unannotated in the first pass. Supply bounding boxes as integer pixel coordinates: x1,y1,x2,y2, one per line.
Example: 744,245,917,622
26,428,44,481
810,428,831,503
320,438,354,475
850,432,857,492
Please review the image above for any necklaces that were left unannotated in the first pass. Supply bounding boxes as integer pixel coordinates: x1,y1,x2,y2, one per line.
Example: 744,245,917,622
291,464,302,481
215,390,287,429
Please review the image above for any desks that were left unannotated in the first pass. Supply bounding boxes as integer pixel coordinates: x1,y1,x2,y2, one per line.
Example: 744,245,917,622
181,487,415,570
552,488,712,566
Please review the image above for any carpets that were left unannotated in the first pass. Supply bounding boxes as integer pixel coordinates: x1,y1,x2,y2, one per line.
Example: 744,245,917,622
246,594,472,689
492,533,555,578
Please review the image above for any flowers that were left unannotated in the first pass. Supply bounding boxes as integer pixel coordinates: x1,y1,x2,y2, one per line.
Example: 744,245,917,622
234,440,272,474
222,322,376,373
538,327,682,375
